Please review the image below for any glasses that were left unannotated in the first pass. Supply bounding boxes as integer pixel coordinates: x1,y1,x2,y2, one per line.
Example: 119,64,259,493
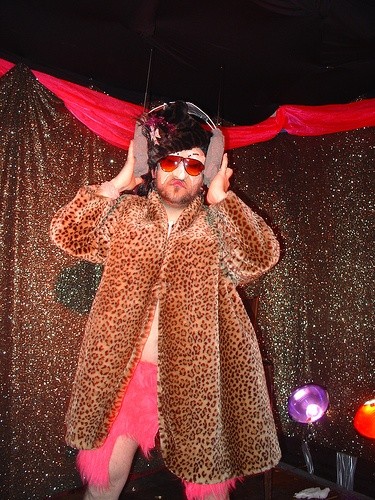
157,155,205,176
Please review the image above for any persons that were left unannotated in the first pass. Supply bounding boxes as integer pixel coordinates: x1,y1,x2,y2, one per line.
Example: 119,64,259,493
48,102,281,500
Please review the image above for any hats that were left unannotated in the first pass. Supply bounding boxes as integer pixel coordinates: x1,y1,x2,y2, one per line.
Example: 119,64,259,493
140,101,212,167
